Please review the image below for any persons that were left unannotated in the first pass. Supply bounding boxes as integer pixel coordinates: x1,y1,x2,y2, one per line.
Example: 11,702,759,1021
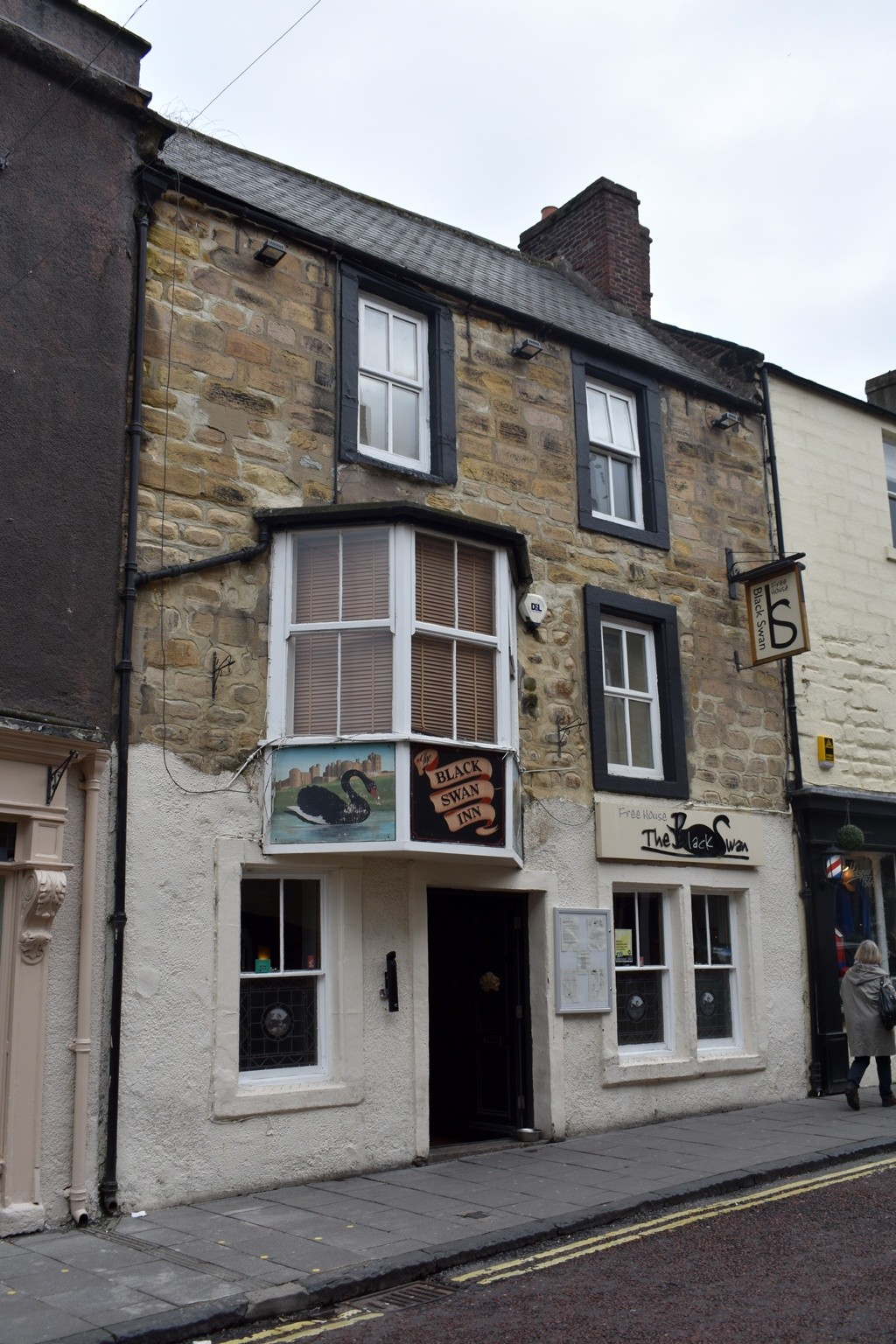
470,971,513,1037
840,940,896,1111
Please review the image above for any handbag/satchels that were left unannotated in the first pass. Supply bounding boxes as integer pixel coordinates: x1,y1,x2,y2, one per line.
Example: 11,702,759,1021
878,976,896,1030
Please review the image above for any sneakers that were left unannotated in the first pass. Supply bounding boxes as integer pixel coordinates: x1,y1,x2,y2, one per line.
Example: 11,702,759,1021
883,1095,896,1107
844,1081,859,1110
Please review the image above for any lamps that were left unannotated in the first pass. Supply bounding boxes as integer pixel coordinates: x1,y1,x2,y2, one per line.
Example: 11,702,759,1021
254,239,288,268
712,413,739,429
512,337,542,361
